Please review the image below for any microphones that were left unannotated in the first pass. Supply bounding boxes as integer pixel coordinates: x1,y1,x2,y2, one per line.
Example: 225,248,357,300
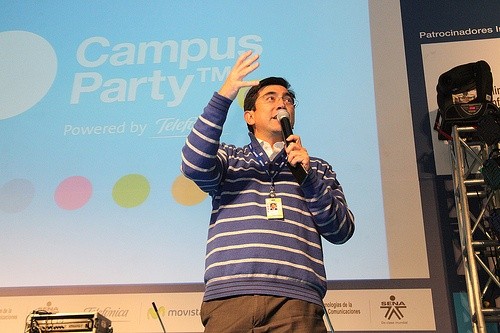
276,109,295,150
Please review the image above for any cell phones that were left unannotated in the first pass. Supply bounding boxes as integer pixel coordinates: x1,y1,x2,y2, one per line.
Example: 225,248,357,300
286,158,308,186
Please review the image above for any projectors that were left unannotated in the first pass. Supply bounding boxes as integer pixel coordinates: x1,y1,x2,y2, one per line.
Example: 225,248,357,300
27,312,112,333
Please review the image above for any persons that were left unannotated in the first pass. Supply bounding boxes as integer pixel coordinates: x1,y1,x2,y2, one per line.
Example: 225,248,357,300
180,50,356,333
